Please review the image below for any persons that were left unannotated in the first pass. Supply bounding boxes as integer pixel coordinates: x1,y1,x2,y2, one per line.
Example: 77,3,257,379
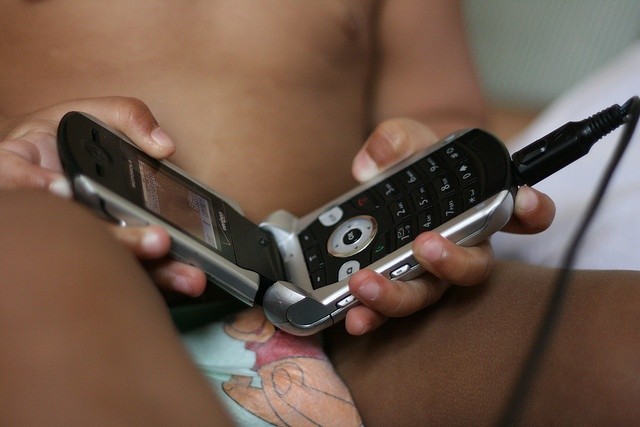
0,0,640,426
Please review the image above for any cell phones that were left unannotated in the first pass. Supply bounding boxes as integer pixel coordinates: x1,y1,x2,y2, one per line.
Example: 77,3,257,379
57,111,516,337
